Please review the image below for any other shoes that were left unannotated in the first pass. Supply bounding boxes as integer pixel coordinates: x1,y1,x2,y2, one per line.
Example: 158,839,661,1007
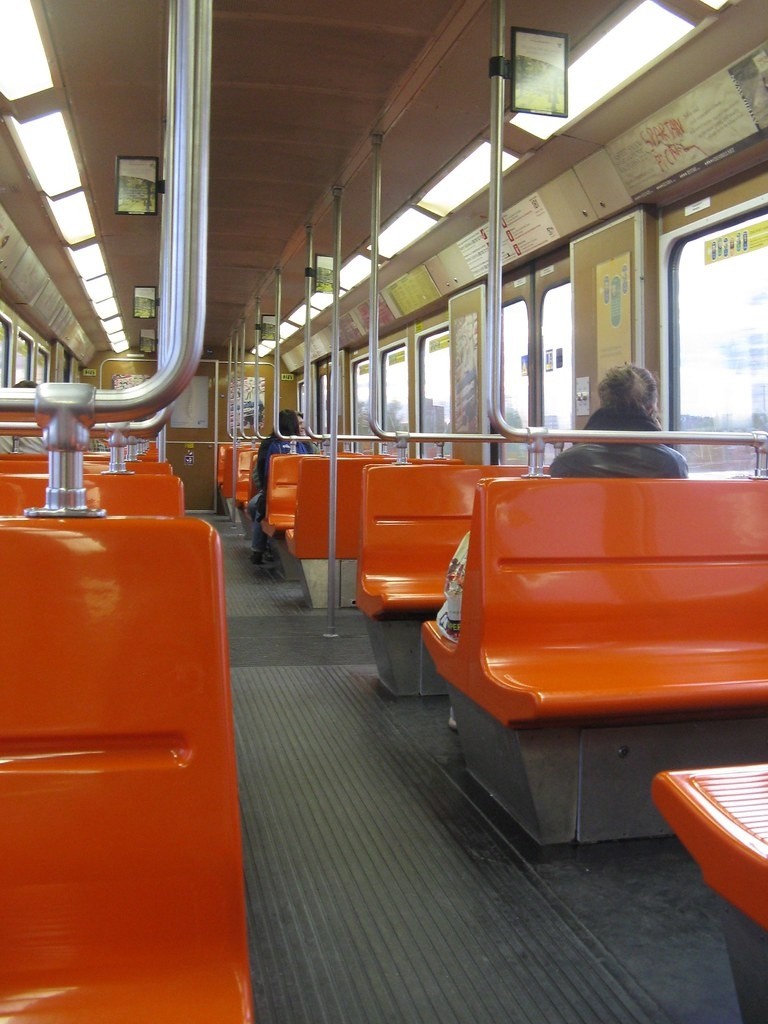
265,548,275,561
250,551,261,564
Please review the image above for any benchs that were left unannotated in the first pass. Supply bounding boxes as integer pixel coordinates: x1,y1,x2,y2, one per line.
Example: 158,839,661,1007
260,454,395,582
0,448,184,518
234,450,259,551
651,765,768,1024
221,447,259,523
285,456,464,609
218,444,261,515
0,516,255,1024
357,464,549,698
422,477,768,846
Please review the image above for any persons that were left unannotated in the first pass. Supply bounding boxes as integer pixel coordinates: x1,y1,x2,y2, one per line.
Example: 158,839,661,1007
248,409,322,564
0,380,48,453
546,361,690,479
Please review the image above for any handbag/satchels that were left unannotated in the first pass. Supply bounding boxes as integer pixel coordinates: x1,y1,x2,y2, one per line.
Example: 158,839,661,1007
434,529,471,643
